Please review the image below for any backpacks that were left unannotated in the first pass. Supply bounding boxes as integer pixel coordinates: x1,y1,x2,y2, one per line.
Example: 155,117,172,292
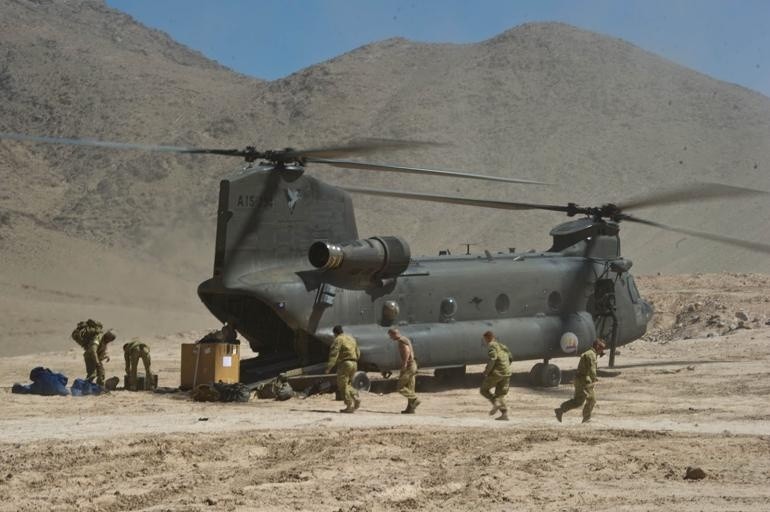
71,318,103,348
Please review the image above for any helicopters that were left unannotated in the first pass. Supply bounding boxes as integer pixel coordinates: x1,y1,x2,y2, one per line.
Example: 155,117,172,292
0,136,770,394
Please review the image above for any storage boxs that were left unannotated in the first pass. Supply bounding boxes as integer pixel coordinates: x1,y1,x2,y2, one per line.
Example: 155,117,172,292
180,343,240,393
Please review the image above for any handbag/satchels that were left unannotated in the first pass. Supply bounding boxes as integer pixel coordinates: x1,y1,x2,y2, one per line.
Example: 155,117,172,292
190,376,294,403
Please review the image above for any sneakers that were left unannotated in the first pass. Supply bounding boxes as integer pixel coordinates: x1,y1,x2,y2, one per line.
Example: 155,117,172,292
400,397,421,414
489,401,509,421
340,395,362,413
554,409,565,423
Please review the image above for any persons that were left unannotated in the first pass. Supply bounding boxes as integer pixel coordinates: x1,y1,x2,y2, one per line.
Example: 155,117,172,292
122,341,155,392
323,324,363,413
387,328,422,413
478,331,513,421
554,339,606,424
81,328,117,387
